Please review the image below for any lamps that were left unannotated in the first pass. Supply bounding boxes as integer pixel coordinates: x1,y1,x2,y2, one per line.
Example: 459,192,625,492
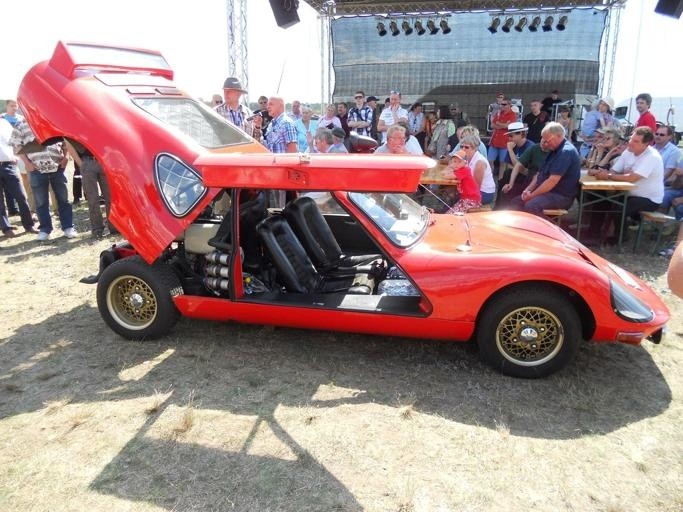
370,11,452,37
485,7,575,34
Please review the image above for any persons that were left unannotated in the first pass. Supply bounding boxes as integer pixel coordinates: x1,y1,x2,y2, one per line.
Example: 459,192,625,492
665,236,683,299
487,88,683,250
0,98,123,242
208,77,498,212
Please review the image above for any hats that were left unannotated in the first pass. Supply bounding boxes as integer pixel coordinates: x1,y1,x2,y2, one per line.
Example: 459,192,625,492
332,127,346,138
600,96,615,111
504,121,529,135
222,77,249,93
367,96,379,102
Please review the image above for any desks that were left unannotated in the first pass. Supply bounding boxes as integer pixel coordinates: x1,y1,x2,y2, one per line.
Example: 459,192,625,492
574,166,639,248
417,158,463,193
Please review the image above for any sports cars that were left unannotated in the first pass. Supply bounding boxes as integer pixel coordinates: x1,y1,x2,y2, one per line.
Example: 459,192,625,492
13,43,675,380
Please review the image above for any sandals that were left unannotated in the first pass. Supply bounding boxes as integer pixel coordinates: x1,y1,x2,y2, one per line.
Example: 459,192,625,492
658,245,675,256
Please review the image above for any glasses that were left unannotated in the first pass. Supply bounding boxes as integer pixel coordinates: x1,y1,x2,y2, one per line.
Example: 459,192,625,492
655,133,665,136
355,96,362,99
460,145,471,149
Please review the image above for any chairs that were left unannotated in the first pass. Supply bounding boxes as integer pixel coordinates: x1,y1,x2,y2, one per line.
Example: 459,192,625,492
256,214,376,296
284,195,390,276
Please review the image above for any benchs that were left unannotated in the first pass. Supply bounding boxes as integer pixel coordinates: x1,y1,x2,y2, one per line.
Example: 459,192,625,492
466,203,491,214
540,208,567,228
632,210,675,256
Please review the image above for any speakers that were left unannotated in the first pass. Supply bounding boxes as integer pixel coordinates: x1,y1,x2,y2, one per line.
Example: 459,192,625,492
654,1,682,20
266,0,300,30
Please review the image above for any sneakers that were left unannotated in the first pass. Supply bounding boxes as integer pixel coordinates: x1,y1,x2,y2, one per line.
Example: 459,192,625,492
64,228,77,238
663,225,672,236
629,222,652,232
25,226,39,233
38,232,48,240
6,230,15,237
